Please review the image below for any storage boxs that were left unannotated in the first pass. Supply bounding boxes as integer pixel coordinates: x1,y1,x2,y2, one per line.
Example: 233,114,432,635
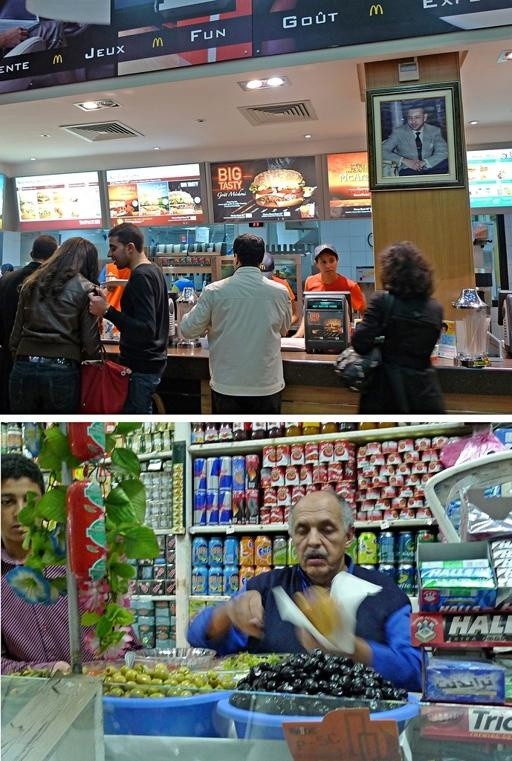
414,539,501,612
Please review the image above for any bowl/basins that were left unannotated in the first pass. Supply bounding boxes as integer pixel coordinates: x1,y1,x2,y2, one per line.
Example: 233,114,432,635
125,647,217,669
215,692,419,741
97,693,228,739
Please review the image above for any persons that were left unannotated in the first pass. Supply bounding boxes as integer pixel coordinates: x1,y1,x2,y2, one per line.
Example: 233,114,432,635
186,489,428,693
1,235,57,414
258,252,299,337
88,223,169,414
8,237,104,414
351,240,447,414
1,264,14,276
99,261,132,333
291,244,368,339
382,106,448,177
180,233,293,415
0,18,67,58
1,453,141,674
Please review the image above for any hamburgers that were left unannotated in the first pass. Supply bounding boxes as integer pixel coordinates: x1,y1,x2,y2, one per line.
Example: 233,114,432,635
169,190,194,215
324,318,342,332
250,168,305,209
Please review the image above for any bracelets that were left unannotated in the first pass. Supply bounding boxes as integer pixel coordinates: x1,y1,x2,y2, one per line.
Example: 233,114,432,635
103,307,112,318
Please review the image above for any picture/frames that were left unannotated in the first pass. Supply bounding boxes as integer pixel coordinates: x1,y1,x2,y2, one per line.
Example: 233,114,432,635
365,80,466,194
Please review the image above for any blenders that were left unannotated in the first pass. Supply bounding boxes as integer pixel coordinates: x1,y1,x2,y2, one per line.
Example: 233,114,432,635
450,290,491,368
174,287,201,348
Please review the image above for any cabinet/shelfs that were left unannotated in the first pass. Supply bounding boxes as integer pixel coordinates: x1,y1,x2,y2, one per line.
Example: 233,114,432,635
1,423,178,649
153,253,302,329
187,423,512,633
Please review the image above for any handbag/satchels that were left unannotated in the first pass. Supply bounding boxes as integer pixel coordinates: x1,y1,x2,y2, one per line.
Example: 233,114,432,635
332,345,382,393
78,354,131,414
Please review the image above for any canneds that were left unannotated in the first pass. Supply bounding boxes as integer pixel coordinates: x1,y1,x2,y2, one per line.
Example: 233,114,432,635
190,454,260,525
259,437,355,522
343,528,434,597
354,431,463,522
190,535,300,596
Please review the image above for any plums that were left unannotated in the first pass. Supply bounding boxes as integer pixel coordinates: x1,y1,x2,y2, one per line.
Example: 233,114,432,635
232,647,407,717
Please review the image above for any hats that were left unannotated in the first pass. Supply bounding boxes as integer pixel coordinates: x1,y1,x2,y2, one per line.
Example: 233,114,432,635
313,244,338,261
259,252,275,277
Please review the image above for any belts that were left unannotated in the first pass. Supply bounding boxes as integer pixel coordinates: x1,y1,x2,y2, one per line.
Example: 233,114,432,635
16,353,76,369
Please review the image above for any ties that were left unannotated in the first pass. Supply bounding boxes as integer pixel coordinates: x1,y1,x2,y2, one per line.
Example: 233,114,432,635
415,131,423,161
